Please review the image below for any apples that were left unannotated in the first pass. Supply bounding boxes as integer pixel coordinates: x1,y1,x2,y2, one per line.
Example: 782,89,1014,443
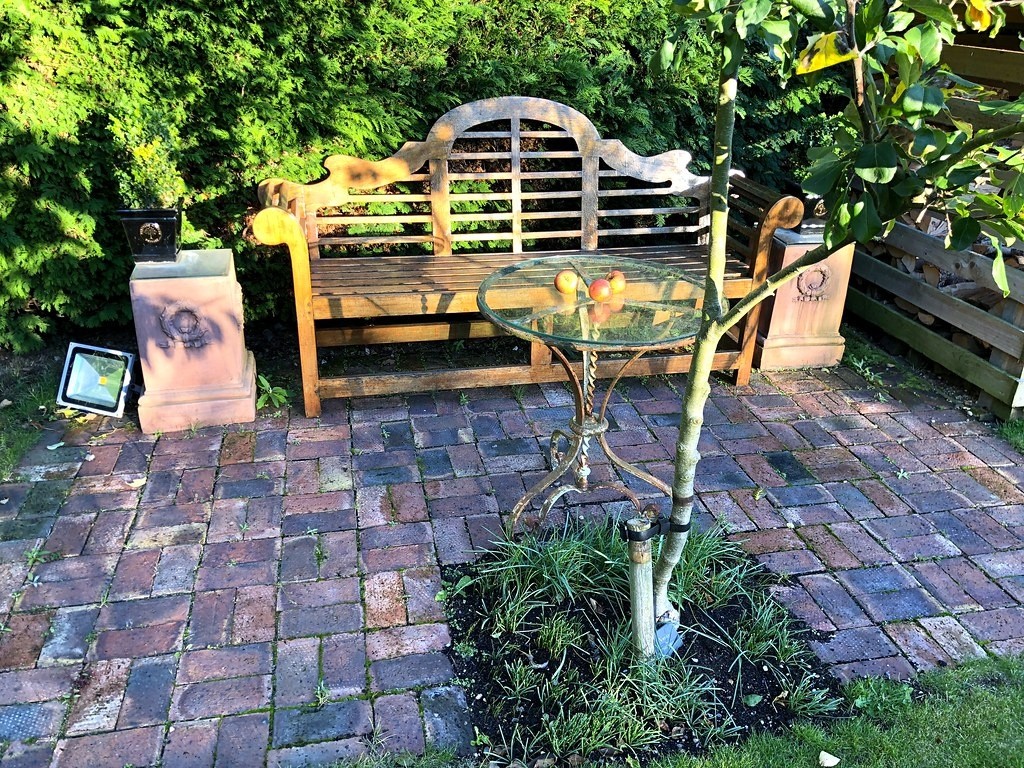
554,270,578,294
588,270,626,302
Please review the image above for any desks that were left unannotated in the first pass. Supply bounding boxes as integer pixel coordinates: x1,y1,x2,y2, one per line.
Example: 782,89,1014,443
476,255,732,541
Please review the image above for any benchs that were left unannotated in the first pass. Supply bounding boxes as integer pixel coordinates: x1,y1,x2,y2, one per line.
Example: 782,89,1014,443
252,97,804,419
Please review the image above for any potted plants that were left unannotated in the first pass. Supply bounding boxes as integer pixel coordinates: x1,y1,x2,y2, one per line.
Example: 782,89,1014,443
113,136,185,263
782,147,848,235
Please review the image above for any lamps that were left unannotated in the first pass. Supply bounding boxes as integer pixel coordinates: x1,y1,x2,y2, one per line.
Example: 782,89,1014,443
56,342,136,419
